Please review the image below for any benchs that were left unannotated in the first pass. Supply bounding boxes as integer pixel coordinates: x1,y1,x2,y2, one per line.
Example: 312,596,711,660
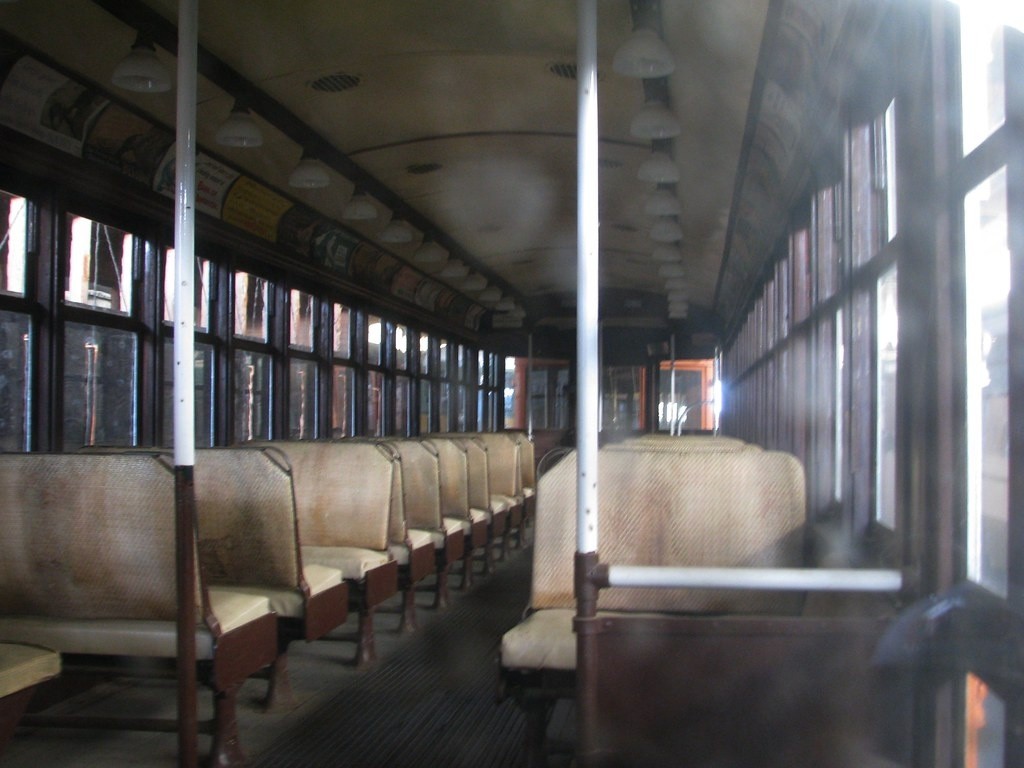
0,429,808,768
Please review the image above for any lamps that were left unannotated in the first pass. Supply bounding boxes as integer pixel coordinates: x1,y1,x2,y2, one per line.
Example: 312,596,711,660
644,183,682,213
610,17,677,79
340,182,378,219
214,97,264,154
650,214,684,242
635,147,681,182
288,144,332,189
413,233,515,311
651,243,688,319
379,206,413,244
110,33,173,96
629,94,681,139
